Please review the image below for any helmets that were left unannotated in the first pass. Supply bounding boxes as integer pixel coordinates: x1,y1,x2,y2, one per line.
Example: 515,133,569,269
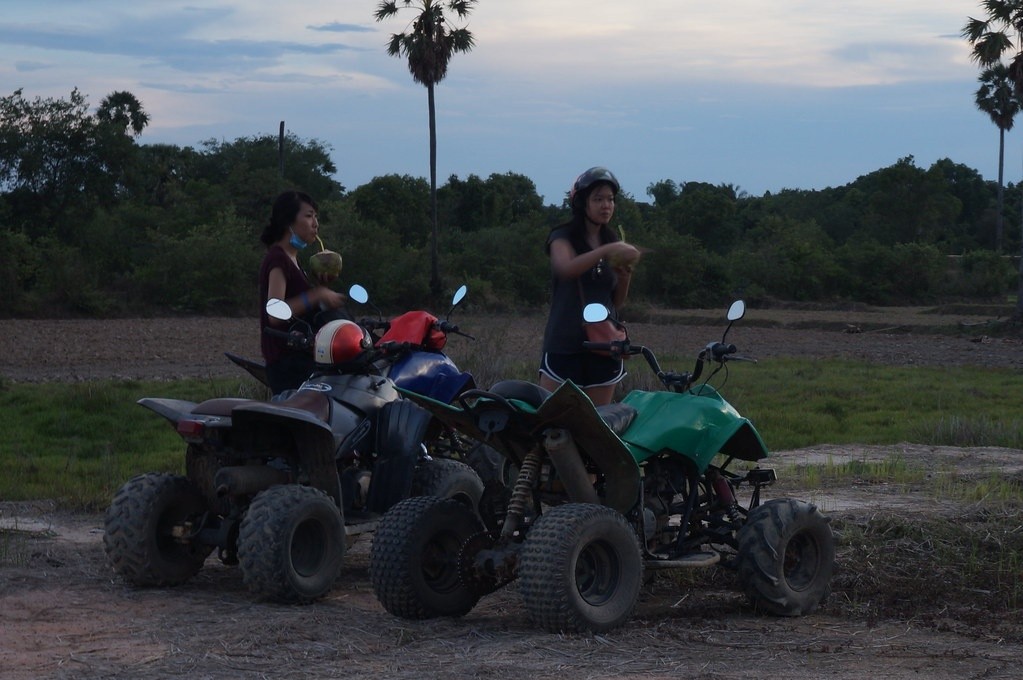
572,166,620,226
313,319,374,366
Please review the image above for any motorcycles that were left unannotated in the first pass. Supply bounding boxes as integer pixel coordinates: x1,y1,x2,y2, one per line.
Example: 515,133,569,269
100,283,480,606
367,297,839,637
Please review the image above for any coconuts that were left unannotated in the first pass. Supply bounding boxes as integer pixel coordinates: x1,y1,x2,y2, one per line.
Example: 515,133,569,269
608,255,637,272
309,250,343,277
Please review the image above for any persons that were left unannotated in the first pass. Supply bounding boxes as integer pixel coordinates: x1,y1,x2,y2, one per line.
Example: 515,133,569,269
536,166,640,407
257,188,352,397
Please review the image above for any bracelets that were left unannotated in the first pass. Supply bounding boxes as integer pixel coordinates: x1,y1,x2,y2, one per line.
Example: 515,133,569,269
301,291,312,310
